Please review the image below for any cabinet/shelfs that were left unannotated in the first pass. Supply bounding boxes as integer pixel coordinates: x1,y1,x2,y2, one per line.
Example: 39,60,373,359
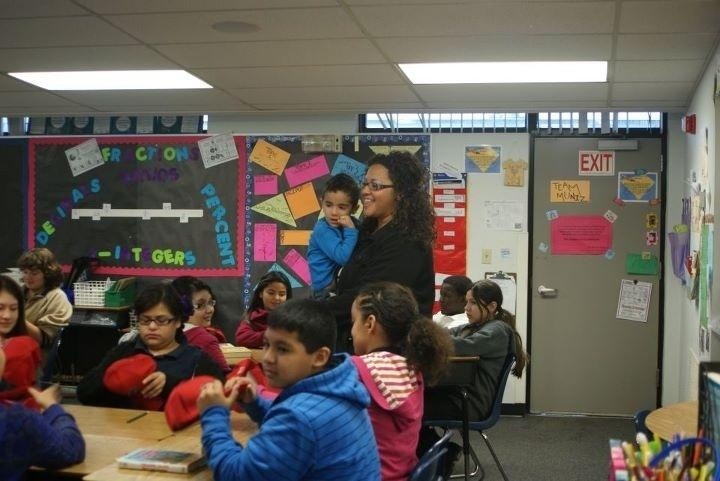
59,304,130,375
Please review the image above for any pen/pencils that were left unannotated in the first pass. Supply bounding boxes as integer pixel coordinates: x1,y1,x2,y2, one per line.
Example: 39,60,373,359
126,412,148,424
621,429,715,481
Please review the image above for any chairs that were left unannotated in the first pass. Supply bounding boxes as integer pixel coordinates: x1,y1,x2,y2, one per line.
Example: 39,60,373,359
424,355,515,481
410,430,454,481
634,409,666,443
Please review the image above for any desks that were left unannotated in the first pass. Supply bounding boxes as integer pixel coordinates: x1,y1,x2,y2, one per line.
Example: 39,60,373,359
227,349,266,365
30,404,260,481
644,400,698,445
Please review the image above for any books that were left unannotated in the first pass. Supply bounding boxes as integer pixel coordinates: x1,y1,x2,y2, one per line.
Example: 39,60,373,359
118,448,206,473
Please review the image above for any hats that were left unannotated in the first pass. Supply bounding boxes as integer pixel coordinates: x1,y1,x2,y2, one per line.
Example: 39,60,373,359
0,336,41,387
102,354,164,412
225,358,268,414
164,376,247,433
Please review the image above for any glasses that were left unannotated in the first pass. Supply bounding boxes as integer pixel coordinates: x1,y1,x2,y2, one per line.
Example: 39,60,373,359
361,182,395,192
138,316,176,327
193,299,216,312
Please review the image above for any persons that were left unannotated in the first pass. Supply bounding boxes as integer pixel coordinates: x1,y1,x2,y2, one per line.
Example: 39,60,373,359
78,283,225,409
304,172,358,302
417,280,526,477
3,338,86,479
175,276,231,373
196,297,381,480
2,275,28,338
432,276,473,329
19,247,74,345
246,281,453,480
233,271,293,349
315,149,439,358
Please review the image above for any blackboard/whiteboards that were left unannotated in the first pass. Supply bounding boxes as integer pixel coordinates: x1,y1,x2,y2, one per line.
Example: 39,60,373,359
0,134,431,348
